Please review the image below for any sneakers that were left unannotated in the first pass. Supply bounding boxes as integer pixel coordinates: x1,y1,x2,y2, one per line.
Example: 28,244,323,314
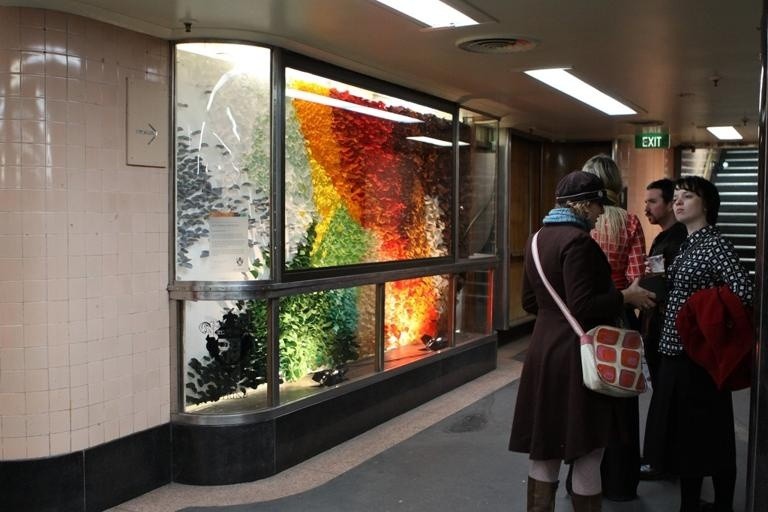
679,500,712,511
638,458,673,482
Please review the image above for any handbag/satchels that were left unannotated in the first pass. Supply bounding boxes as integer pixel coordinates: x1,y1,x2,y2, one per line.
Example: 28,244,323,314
581,326,648,397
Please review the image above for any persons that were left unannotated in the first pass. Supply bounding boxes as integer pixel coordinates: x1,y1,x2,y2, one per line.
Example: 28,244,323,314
642,175,760,511
637,177,688,482
564,153,651,506
506,169,659,511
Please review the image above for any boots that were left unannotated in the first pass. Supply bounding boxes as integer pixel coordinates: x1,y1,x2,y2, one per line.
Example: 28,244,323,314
572,490,600,510
527,476,559,511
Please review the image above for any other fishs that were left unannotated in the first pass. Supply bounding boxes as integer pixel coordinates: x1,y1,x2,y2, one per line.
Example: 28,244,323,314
174,86,361,409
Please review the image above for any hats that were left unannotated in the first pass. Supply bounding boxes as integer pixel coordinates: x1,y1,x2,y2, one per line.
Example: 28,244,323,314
556,171,616,206
